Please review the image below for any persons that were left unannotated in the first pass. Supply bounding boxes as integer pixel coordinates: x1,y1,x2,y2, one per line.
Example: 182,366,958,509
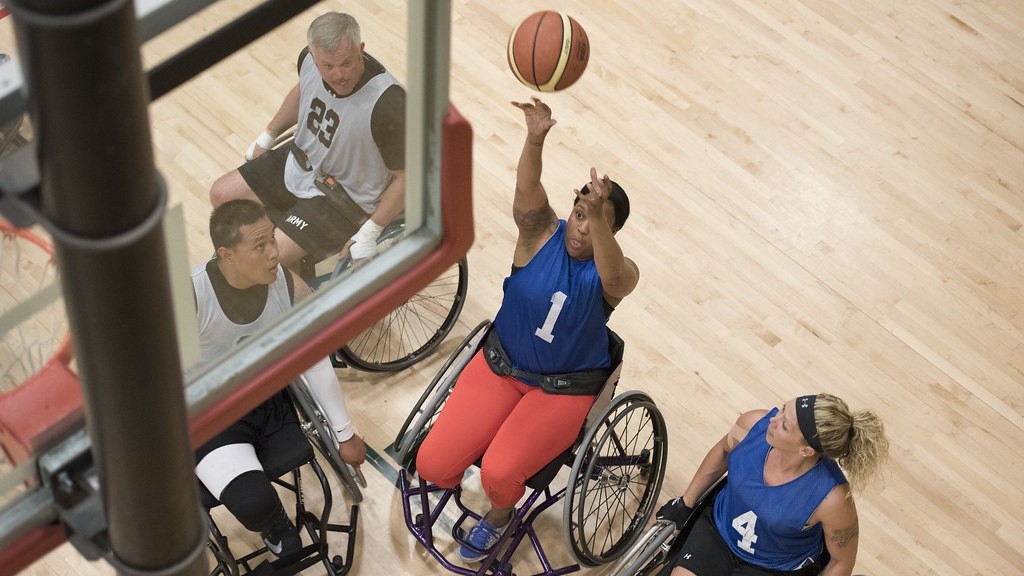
416,92,639,569
656,392,890,576
189,199,366,564
210,11,407,278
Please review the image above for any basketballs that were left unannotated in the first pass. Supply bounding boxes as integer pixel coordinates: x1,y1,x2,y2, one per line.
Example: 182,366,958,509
507,7,592,94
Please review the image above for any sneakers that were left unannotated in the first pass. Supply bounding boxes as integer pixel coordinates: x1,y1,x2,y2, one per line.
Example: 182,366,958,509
459,509,517,566
260,520,303,561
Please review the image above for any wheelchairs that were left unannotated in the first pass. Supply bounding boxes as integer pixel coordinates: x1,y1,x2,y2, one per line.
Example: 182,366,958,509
604,469,735,576
202,375,369,576
387,315,669,576
258,127,471,376
